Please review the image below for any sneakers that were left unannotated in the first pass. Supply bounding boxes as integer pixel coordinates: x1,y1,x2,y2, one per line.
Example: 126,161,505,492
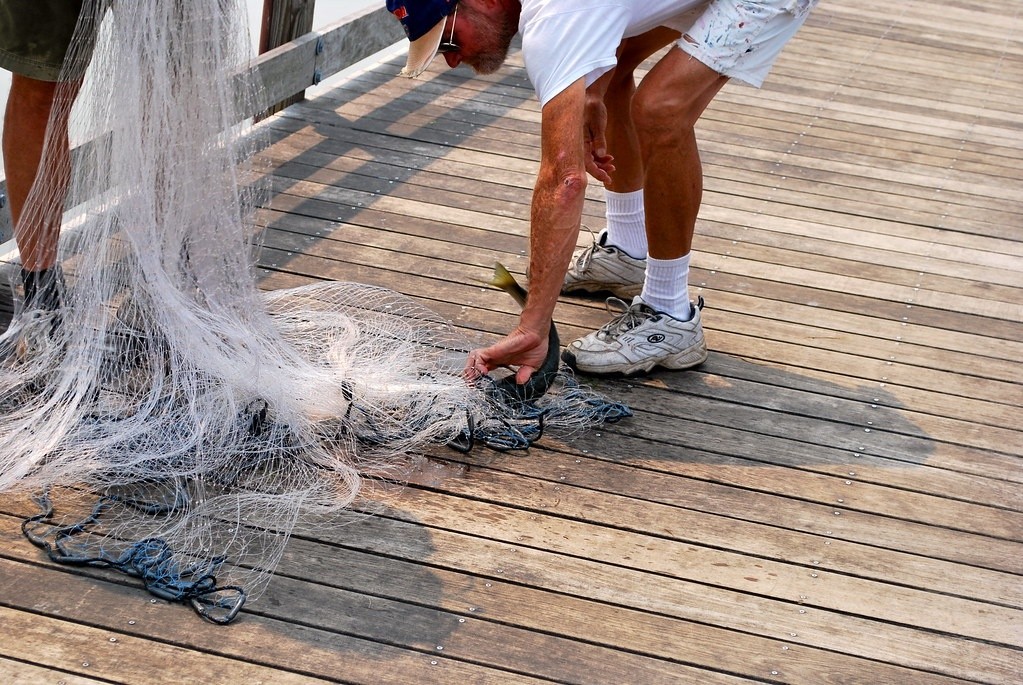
525,224,646,300
561,296,707,376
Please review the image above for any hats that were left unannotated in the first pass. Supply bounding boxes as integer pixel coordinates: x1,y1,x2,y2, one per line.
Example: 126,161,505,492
386,0,459,77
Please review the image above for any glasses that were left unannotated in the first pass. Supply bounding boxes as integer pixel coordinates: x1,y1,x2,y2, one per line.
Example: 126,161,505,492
436,2,460,53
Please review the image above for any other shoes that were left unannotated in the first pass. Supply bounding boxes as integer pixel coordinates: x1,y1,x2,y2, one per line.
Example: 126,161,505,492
116,293,172,335
19,316,100,403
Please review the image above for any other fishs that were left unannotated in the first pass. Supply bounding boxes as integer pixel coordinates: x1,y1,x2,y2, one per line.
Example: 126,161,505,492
478,262,560,409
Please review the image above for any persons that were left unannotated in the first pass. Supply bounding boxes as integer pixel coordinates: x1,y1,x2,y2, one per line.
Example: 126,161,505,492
0,0,230,395
384,0,817,386
